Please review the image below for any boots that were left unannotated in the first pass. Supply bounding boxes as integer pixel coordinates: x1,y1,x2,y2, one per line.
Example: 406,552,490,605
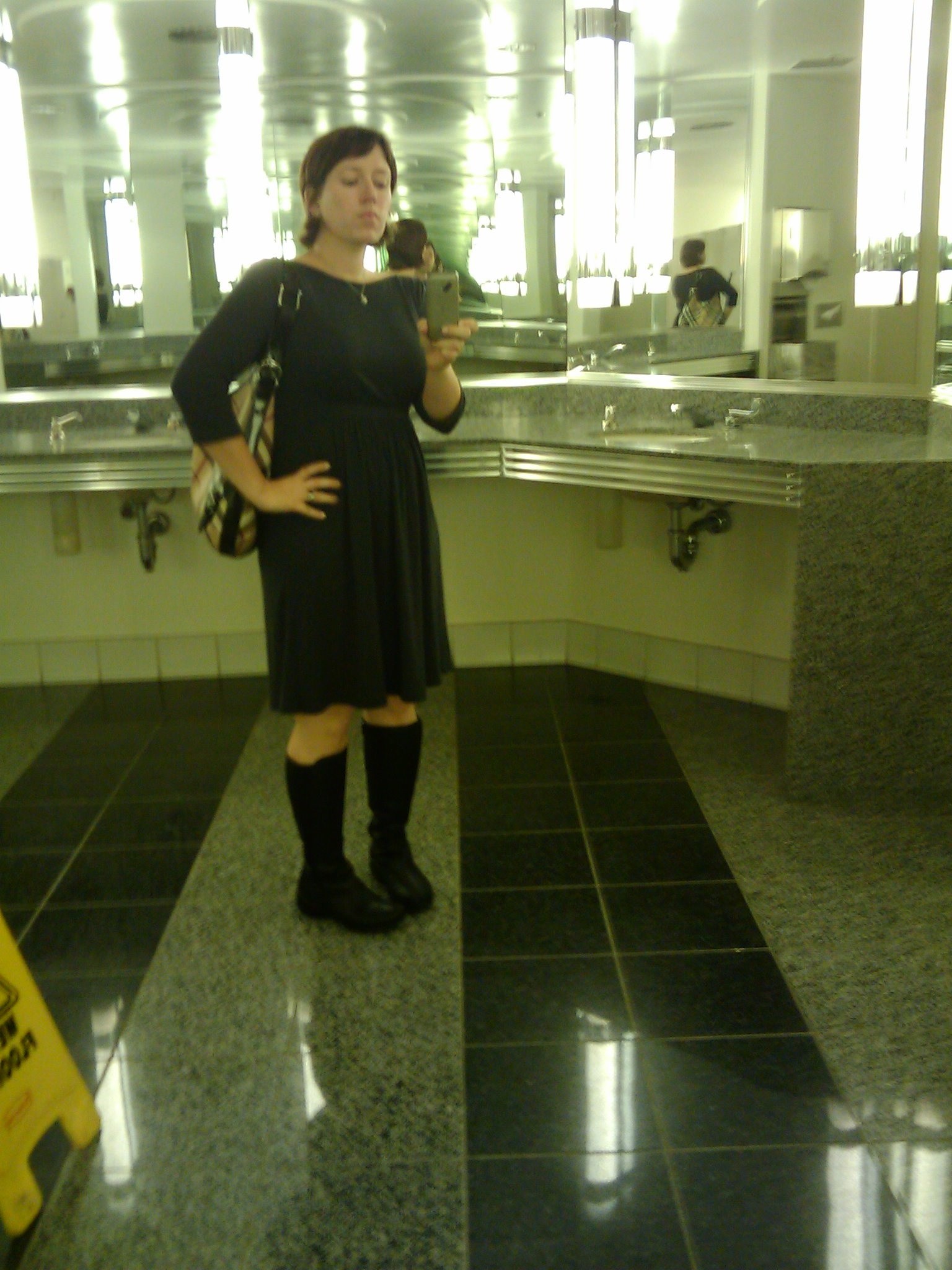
285,744,398,932
363,713,436,909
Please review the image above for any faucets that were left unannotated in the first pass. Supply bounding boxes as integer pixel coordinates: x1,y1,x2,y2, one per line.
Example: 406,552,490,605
127,411,154,432
600,344,626,363
670,401,718,428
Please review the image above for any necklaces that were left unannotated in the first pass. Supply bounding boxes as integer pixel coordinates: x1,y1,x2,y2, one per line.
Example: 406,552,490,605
313,248,369,304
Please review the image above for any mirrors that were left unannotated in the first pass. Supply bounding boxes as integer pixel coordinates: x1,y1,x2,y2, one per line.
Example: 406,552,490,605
0,0,952,394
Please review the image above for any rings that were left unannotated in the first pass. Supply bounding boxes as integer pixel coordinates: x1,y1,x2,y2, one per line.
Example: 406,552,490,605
307,489,315,503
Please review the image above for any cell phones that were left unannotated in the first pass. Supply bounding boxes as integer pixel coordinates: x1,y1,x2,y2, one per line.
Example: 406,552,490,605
426,272,458,340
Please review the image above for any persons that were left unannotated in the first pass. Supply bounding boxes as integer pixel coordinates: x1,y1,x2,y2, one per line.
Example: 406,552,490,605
671,238,738,327
168,127,482,931
94,266,112,326
382,216,443,286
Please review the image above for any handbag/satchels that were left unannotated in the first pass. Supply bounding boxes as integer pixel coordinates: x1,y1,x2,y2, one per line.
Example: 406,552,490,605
191,260,302,558
675,272,723,328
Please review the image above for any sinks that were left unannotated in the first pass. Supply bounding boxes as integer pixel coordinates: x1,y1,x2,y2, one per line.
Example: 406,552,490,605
64,434,178,447
586,361,630,371
589,425,717,446
